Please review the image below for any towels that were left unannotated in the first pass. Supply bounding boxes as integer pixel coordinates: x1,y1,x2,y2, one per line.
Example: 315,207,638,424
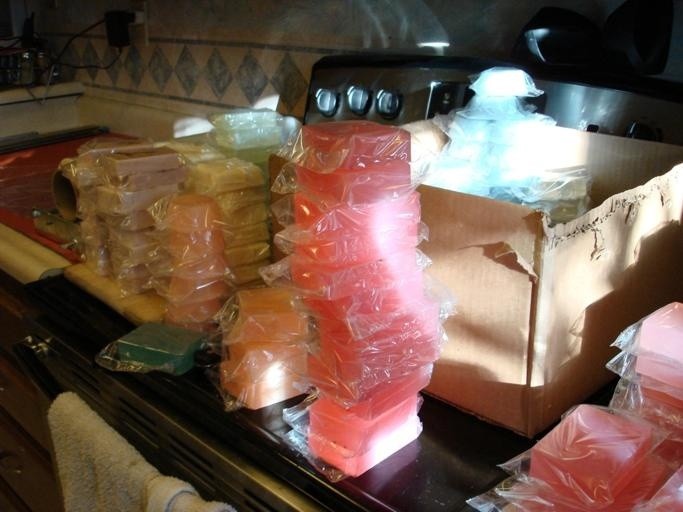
47,391,235,512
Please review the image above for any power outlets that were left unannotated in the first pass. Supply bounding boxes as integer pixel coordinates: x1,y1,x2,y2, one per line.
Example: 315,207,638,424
128,0,148,47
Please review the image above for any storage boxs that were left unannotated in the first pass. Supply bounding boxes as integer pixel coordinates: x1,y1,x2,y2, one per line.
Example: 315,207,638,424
379,112,683,440
